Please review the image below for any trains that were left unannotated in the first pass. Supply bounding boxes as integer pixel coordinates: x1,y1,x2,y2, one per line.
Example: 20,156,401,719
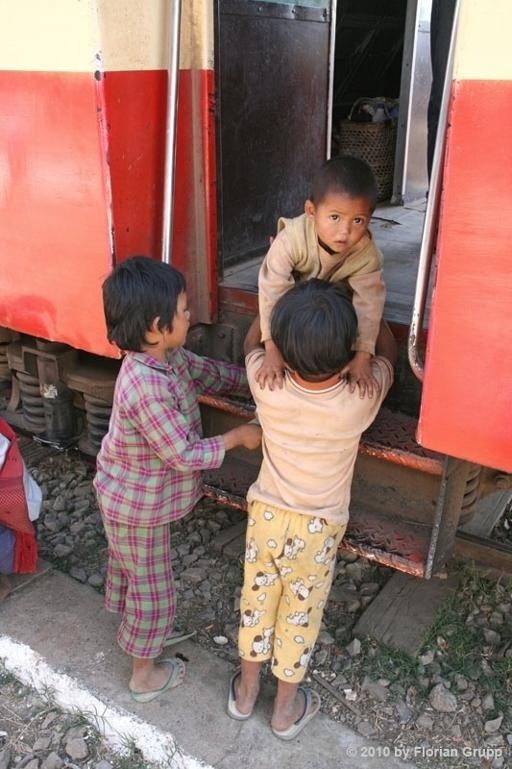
0,1,512,582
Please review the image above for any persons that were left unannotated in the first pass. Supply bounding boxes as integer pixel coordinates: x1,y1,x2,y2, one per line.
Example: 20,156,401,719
0,413,44,597
225,278,400,745
93,256,262,705
254,155,387,400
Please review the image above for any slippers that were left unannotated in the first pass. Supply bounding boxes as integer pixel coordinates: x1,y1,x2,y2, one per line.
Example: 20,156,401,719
272,686,321,741
162,629,197,648
226,668,253,721
130,658,185,703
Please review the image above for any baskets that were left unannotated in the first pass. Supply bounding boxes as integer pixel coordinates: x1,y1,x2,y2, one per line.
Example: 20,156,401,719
340,97,397,203
